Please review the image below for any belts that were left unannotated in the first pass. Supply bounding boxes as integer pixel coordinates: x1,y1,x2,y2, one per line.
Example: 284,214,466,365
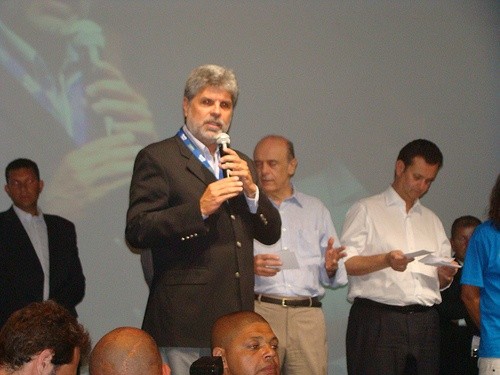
255,295,321,307
355,298,438,314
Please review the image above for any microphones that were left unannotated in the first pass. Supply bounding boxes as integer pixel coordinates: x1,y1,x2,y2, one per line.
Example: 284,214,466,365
215,133,238,208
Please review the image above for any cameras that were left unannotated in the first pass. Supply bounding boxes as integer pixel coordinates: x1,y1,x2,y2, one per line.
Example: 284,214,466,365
189,356,224,375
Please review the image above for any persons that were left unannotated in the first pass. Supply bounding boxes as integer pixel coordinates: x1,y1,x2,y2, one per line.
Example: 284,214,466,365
433,171,500,375
339,138,459,375
210,310,280,375
0,159,85,375
253,135,348,375
0,300,171,375
124,64,282,375
